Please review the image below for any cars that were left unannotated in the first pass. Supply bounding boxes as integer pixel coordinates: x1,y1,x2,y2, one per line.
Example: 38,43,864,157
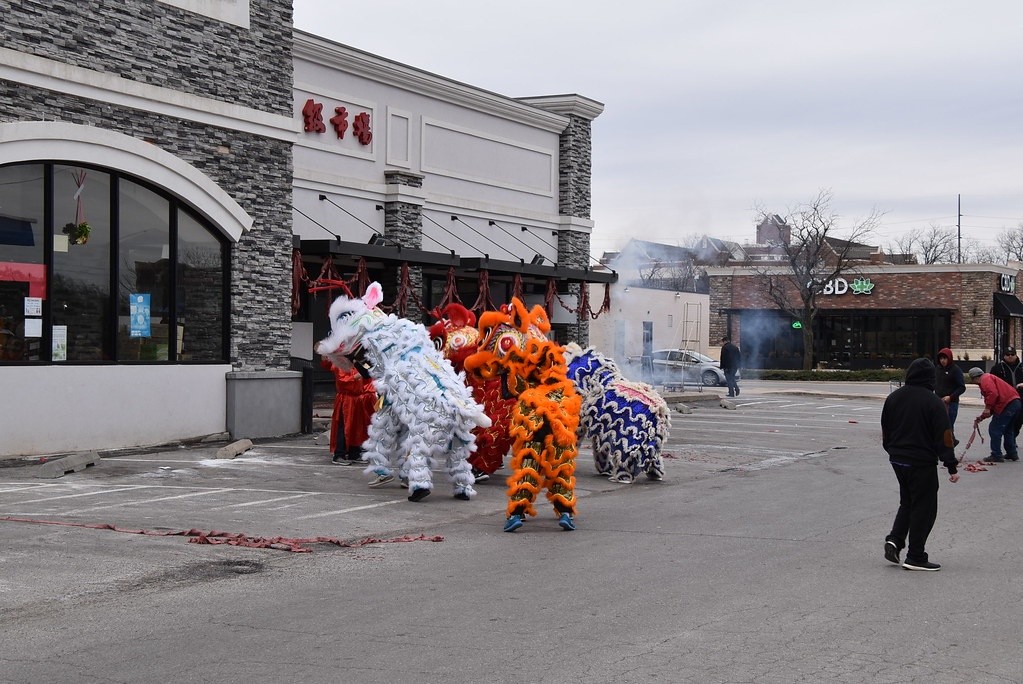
626,349,741,386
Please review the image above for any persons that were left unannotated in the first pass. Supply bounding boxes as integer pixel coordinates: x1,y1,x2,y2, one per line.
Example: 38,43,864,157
641,335,655,376
879,359,959,570
933,348,967,469
990,347,1023,447
720,337,742,397
968,367,1023,461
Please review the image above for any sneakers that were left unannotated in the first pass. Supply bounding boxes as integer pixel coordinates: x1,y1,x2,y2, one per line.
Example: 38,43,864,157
473,470,490,483
454,493,470,500
519,513,526,522
400,477,408,488
902,558,942,571
498,461,504,469
883,541,901,564
559,514,575,530
348,455,369,464
332,457,351,466
368,474,394,487
983,455,1004,462
1003,453,1019,461
408,489,430,502
504,515,522,532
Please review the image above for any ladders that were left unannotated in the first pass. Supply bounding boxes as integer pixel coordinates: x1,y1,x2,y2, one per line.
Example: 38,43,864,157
661,302,703,394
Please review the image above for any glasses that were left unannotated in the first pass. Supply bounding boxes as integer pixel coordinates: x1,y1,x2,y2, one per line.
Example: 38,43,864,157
1005,352,1016,356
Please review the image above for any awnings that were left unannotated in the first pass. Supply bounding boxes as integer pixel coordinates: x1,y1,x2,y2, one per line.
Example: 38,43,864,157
994,292,1023,316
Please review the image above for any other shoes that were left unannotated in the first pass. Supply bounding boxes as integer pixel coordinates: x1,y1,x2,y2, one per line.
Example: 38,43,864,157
649,475,662,481
726,394,734,397
618,479,631,483
735,389,740,396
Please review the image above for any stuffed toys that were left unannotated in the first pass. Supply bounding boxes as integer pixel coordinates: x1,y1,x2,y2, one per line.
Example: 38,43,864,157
311,280,671,535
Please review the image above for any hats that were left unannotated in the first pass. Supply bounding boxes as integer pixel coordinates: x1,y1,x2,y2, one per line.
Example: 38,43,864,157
969,367,984,378
1003,346,1016,354
